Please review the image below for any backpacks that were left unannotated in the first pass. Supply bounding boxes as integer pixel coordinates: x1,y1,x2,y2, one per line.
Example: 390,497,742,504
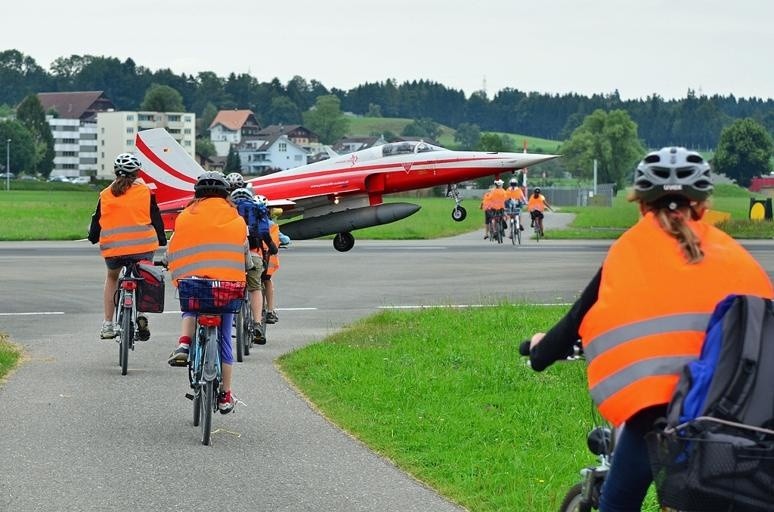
236,200,269,240
670,294,774,512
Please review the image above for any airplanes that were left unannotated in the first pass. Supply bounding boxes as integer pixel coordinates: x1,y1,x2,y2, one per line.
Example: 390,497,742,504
133,127,564,252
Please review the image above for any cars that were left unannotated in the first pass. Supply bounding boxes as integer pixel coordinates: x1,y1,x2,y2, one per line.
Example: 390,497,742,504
455,178,479,190
0,173,92,186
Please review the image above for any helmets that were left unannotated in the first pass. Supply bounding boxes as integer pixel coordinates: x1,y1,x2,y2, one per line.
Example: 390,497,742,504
634,147,713,199
114,153,142,172
194,171,268,205
510,178,518,184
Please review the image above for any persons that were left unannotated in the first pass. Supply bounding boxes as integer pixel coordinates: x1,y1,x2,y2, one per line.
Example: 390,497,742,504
87,152,167,342
161,166,280,414
525,144,773,512
480,175,553,243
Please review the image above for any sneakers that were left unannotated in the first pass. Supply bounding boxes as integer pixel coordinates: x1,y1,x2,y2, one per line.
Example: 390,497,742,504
135,313,150,341
266,311,278,324
99,322,114,338
254,322,266,345
167,343,189,363
218,393,238,415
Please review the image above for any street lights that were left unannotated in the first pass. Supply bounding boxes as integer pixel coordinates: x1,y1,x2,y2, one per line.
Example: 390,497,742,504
7,139,10,191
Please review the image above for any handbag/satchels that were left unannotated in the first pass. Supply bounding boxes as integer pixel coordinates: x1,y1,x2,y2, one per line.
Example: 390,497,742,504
133,260,163,294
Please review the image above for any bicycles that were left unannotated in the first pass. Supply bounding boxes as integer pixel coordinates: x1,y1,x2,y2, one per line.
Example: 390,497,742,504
479,201,528,245
152,257,256,445
89,230,167,376
232,239,293,361
529,207,547,242
518,333,772,511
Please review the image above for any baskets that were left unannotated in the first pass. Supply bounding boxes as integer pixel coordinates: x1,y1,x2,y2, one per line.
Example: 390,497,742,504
176,278,246,314
137,281,165,313
642,432,774,512
